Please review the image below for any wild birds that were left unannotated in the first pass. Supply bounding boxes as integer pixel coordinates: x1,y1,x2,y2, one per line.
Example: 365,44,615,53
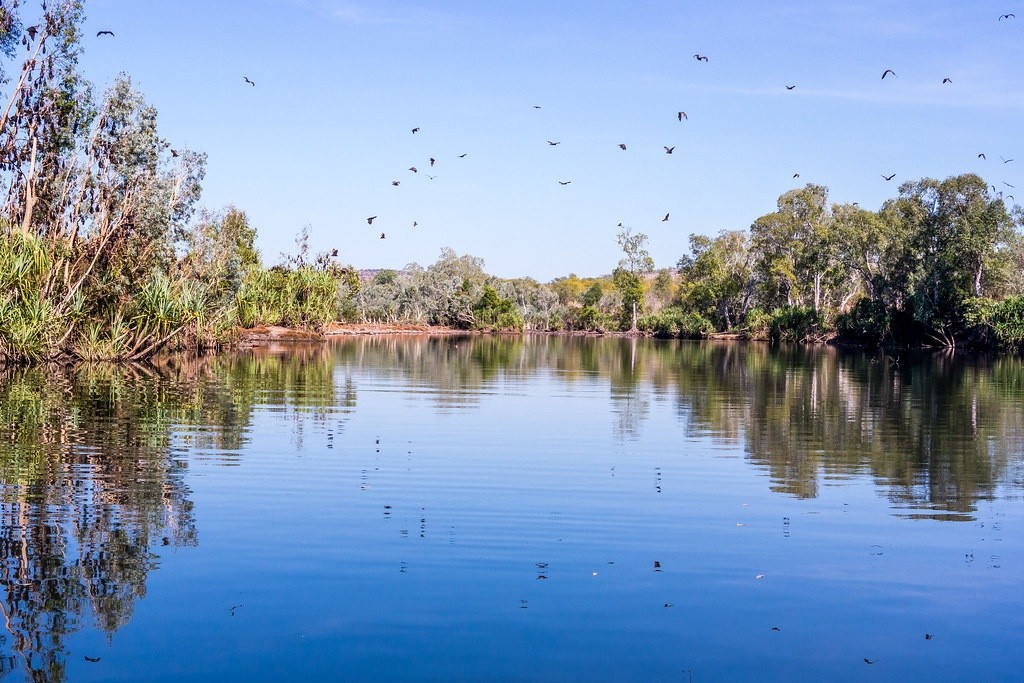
96,31,114,37
693,55,709,62
853,202,858,205
27,26,39,42
793,173,800,178
662,213,669,222
617,223,623,226
786,85,796,89
881,173,896,180
943,78,953,84
244,76,255,86
881,69,896,79
978,154,1016,202
998,14,1016,21
534,105,688,188
331,127,467,258
